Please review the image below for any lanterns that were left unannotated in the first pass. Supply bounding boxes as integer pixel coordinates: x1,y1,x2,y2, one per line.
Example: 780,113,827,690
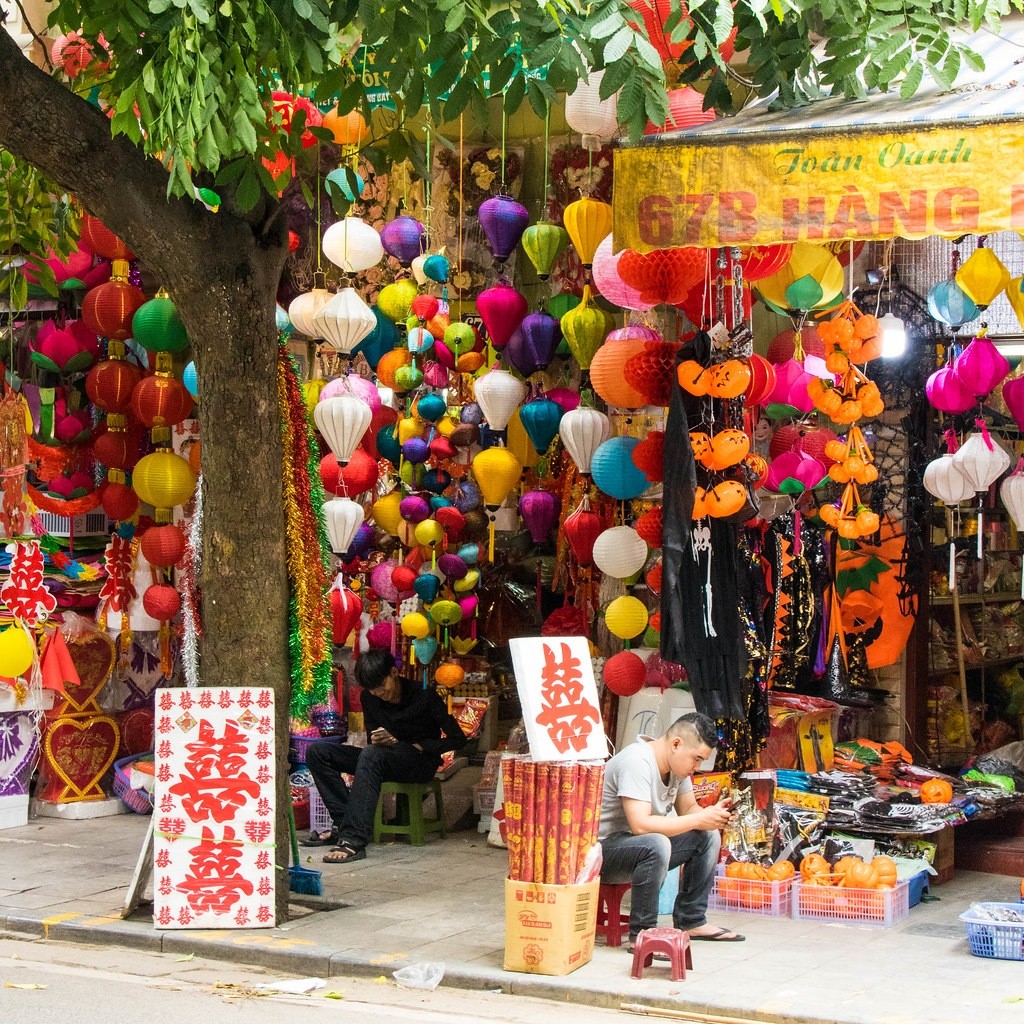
3,2,1024,919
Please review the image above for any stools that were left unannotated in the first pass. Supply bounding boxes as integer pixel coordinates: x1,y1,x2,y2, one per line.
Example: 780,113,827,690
595,883,632,948
631,927,693,982
374,777,450,846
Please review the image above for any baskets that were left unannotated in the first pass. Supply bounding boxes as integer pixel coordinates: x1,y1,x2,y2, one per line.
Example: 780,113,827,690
309,786,338,834
909,870,928,908
791,878,910,927
112,751,155,814
292,800,310,829
959,902,1024,960
707,864,801,916
290,735,347,762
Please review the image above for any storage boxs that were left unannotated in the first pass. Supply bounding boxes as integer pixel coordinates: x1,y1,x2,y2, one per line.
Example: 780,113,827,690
0,794,29,830
958,901,1024,961
289,735,347,764
309,787,334,835
503,875,601,976
790,878,910,928
909,869,930,908
111,751,155,815
706,871,800,918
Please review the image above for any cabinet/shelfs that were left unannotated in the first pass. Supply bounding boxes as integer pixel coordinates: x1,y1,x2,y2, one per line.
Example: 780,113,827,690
928,425,1024,772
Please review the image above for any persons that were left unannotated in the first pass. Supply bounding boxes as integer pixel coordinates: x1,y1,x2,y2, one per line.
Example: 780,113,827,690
306,648,467,865
595,713,746,962
